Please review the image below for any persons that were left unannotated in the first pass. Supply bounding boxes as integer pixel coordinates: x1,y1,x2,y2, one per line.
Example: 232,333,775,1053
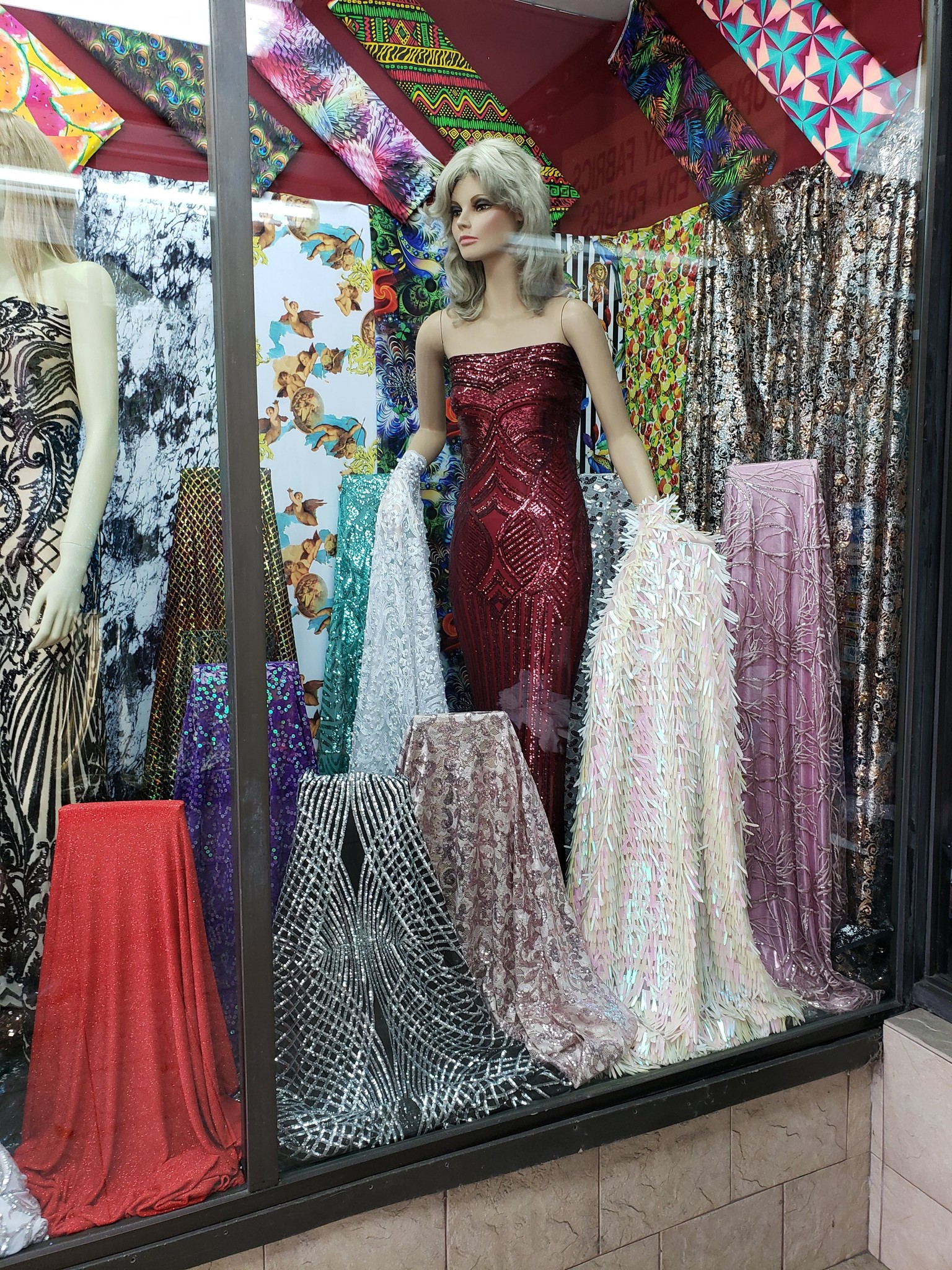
0,112,120,1064
403,136,658,880
